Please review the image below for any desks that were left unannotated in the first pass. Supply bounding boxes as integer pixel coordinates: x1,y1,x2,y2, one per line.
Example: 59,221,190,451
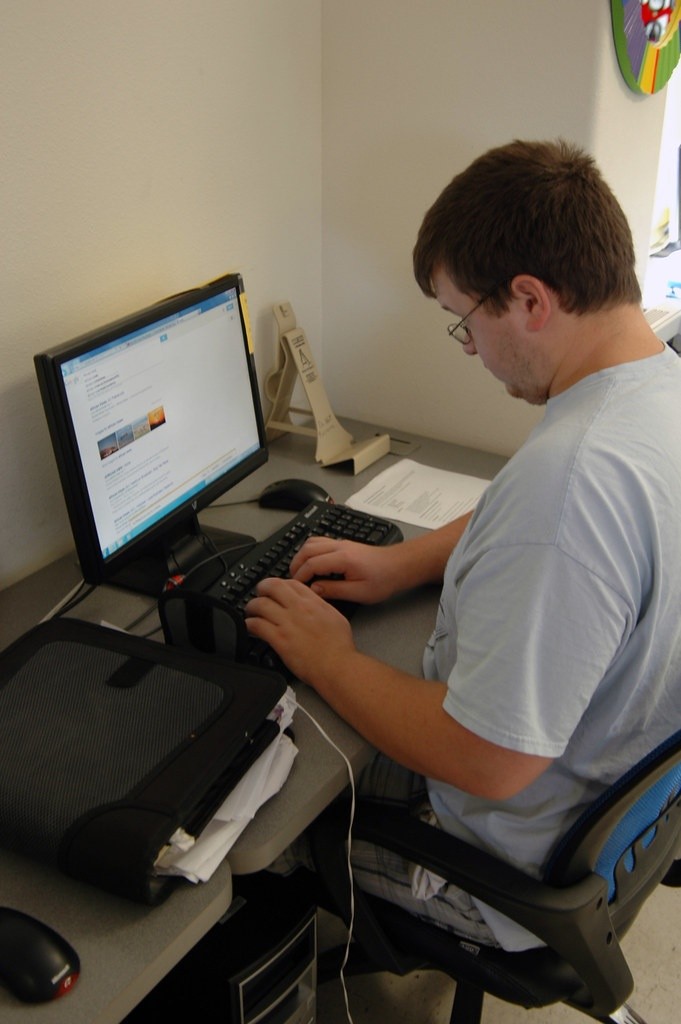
0,416,508,1024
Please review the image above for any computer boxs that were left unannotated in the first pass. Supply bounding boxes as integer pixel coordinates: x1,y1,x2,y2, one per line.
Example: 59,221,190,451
148,879,318,1024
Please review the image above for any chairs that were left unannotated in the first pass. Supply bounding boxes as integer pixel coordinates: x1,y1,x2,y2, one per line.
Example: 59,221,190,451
294,734,681,1024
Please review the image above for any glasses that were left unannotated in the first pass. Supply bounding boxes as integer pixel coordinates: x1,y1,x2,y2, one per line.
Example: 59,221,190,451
444,279,506,344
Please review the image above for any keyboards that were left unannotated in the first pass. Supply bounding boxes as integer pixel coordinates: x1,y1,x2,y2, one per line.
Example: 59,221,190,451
204,500,404,676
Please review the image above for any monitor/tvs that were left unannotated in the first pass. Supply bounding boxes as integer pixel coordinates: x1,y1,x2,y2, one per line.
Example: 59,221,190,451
33,270,269,596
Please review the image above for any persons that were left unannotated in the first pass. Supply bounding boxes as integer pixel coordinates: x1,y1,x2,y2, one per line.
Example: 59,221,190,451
244,140,681,952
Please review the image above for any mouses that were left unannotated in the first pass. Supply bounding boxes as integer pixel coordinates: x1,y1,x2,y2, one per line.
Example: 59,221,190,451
259,477,334,511
0,906,79,1004
